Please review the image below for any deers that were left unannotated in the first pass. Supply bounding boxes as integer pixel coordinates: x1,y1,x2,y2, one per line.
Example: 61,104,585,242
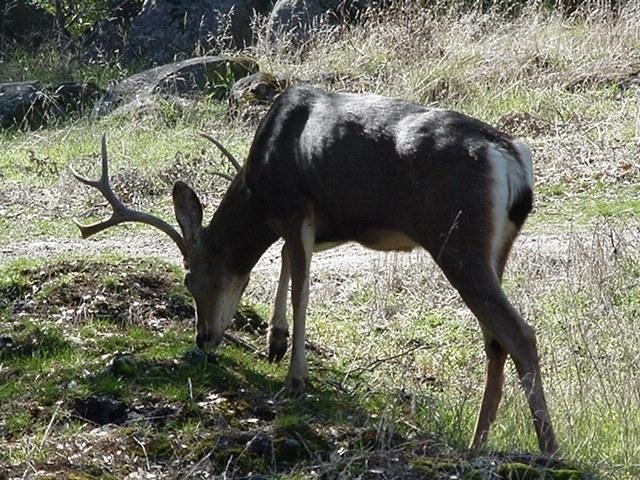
67,84,564,469
